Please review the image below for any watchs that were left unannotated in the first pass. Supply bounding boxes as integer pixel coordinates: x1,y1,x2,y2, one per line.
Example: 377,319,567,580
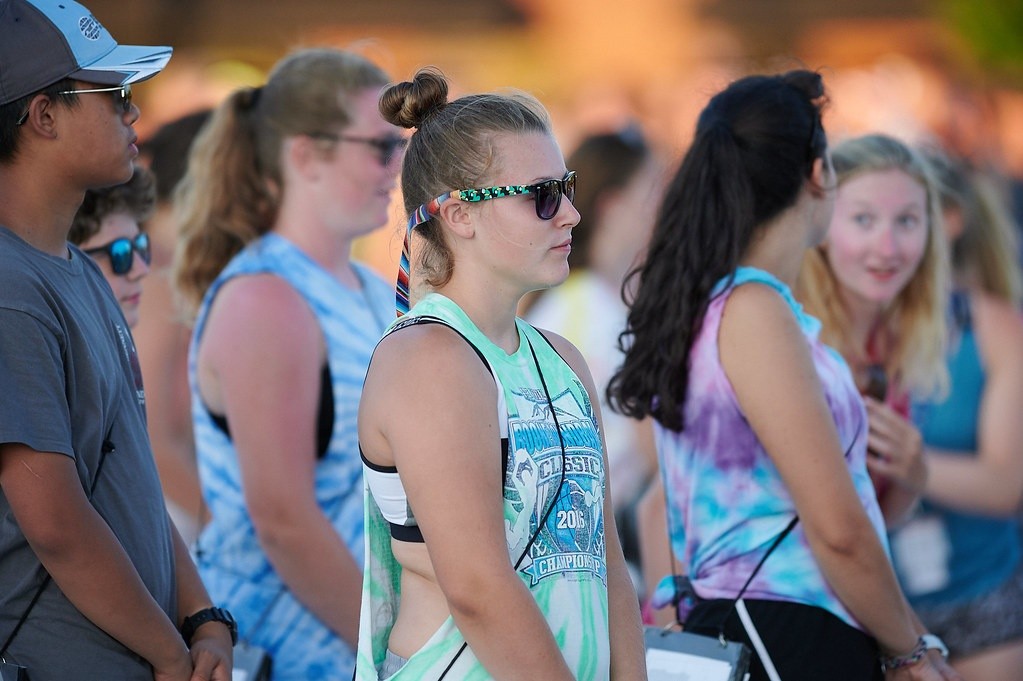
179,607,238,650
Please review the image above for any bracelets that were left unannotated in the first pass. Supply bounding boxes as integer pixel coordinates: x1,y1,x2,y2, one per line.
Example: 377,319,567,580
923,633,949,661
883,636,927,670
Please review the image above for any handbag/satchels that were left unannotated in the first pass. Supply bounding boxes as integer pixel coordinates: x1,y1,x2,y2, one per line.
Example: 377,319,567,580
641,625,749,681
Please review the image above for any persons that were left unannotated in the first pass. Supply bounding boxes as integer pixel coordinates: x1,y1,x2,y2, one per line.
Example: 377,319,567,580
171,49,406,681
68,166,159,329
521,119,673,628
790,133,949,534
0,0,242,681
358,64,647,681
883,139,1023,680
605,70,959,681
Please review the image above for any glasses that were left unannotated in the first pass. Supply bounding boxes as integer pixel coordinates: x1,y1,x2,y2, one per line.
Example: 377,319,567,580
459,170,577,220
311,132,407,165
83,232,151,274
16,84,132,126
777,69,824,177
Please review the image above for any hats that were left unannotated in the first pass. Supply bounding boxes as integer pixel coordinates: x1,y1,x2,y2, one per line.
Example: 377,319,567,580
0,0,173,105
